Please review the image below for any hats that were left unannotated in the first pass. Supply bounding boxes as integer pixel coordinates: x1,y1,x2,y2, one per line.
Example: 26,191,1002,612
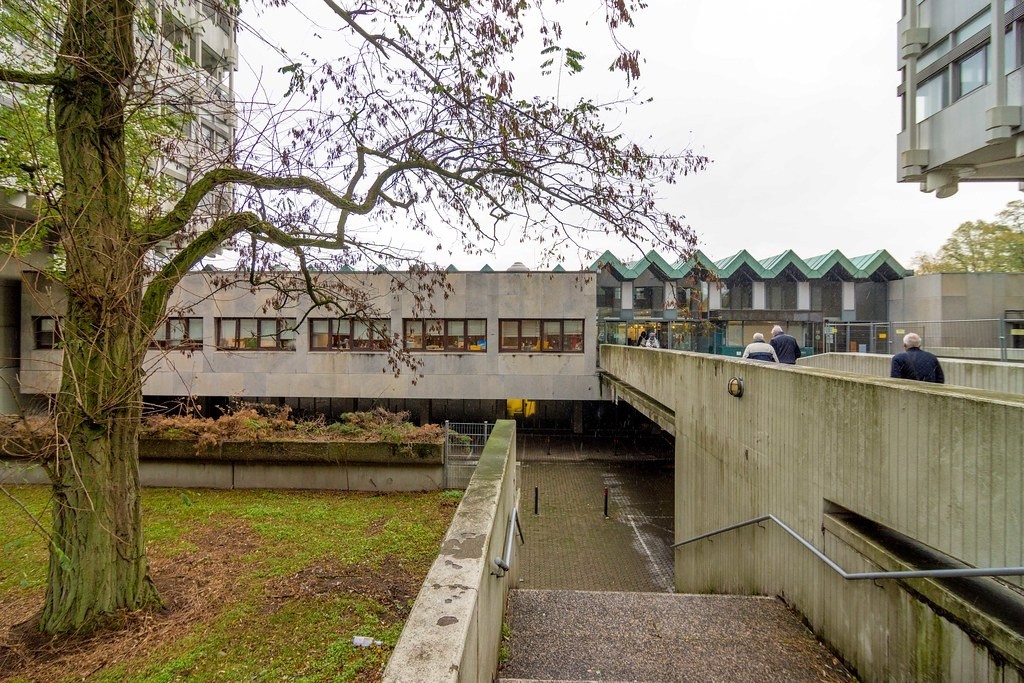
649,332,655,336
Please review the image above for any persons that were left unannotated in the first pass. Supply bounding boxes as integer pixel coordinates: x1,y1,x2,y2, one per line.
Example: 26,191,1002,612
890,332,944,385
742,333,779,363
646,332,661,348
637,331,649,347
769,325,801,365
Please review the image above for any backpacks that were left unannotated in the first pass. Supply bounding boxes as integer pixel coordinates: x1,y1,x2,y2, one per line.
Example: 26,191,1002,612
640,336,646,346
